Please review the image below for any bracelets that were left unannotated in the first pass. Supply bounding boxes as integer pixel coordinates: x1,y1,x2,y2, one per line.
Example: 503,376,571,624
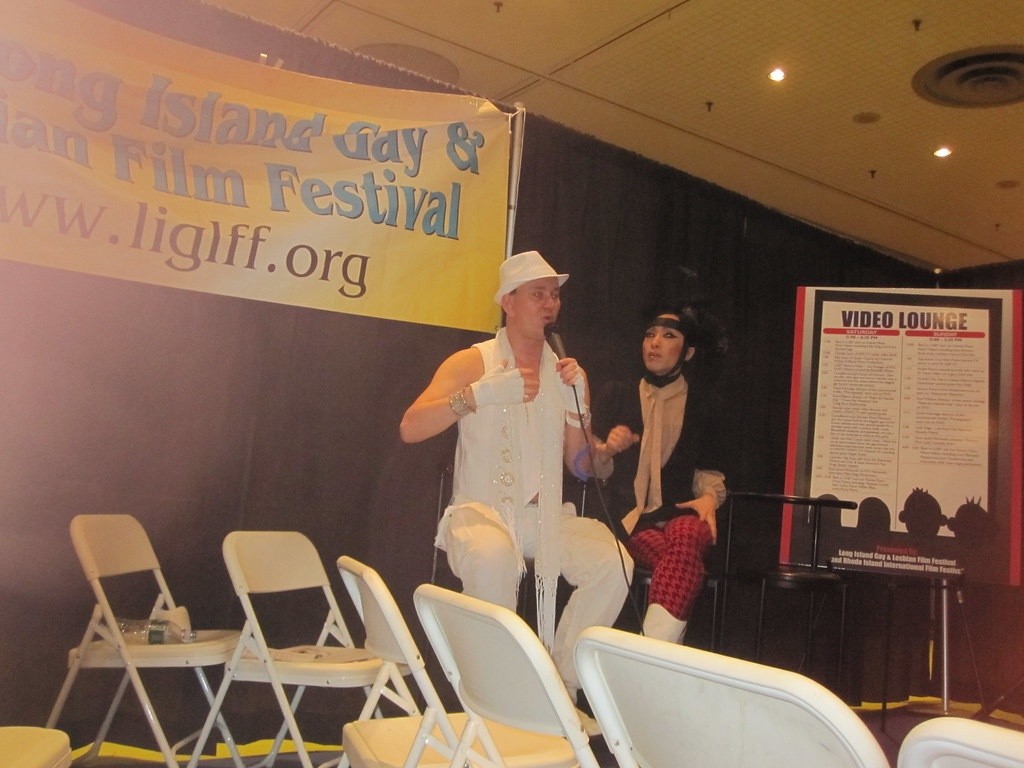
448,388,476,419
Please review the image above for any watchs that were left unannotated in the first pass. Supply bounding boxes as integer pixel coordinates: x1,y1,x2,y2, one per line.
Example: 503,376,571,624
565,406,592,430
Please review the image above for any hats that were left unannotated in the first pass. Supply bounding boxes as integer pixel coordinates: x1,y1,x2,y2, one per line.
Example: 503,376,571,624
494,251,569,305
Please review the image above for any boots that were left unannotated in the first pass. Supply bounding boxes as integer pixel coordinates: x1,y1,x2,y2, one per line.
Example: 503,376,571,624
640,603,687,645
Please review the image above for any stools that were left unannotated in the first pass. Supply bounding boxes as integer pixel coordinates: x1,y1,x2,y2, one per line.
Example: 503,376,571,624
430,459,860,699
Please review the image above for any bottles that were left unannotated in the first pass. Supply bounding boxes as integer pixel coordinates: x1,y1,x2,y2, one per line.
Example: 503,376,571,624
105,617,197,645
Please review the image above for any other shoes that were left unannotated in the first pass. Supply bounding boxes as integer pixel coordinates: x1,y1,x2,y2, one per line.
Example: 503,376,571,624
574,705,602,737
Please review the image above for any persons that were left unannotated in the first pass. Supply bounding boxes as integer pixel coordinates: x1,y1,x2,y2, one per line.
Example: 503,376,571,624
399,251,635,736
589,302,728,646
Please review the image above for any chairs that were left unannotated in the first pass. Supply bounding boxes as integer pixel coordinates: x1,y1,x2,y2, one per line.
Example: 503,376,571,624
1,724,74,768
895,717,1024,767
413,583,603,768
574,625,892,768
44,512,247,768
187,530,413,768
335,555,589,768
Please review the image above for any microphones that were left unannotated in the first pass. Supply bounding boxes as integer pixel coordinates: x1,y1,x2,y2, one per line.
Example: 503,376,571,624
543,322,576,388
